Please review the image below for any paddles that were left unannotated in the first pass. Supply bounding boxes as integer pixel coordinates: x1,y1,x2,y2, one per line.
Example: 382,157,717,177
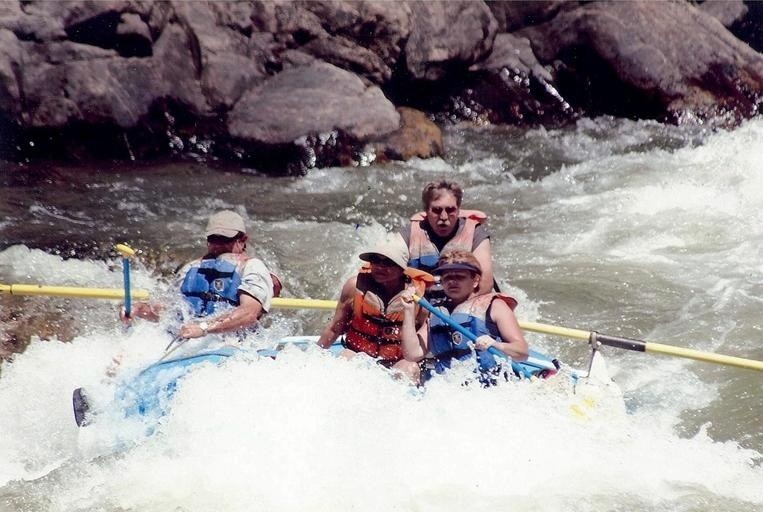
405,290,597,418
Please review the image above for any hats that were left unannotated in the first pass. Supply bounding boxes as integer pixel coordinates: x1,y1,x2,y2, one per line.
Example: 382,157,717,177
204,208,248,239
432,263,483,274
359,230,411,269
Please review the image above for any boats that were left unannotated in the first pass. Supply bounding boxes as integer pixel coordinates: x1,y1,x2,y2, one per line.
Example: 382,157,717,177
107,337,589,432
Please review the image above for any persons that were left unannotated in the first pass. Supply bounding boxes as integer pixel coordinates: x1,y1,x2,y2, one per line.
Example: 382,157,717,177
399,179,493,297
314,242,433,386
117,209,270,339
393,249,528,388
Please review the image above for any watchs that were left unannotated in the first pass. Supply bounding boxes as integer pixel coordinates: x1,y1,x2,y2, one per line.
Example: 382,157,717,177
197,318,209,336
